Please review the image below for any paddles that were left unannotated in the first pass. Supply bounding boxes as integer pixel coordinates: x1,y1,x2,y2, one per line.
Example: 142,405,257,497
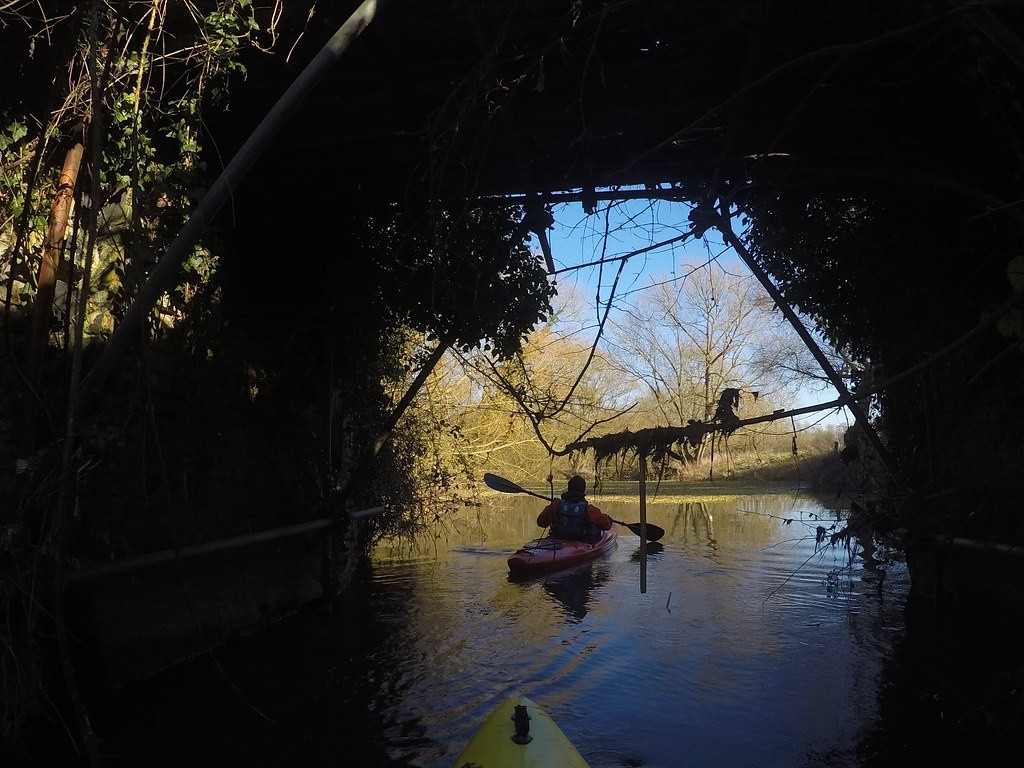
484,473,664,542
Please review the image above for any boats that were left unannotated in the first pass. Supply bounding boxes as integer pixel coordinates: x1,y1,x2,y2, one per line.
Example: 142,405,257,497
507,522,619,577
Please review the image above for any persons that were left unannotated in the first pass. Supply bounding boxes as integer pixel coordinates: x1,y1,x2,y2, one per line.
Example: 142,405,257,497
536,475,613,545
